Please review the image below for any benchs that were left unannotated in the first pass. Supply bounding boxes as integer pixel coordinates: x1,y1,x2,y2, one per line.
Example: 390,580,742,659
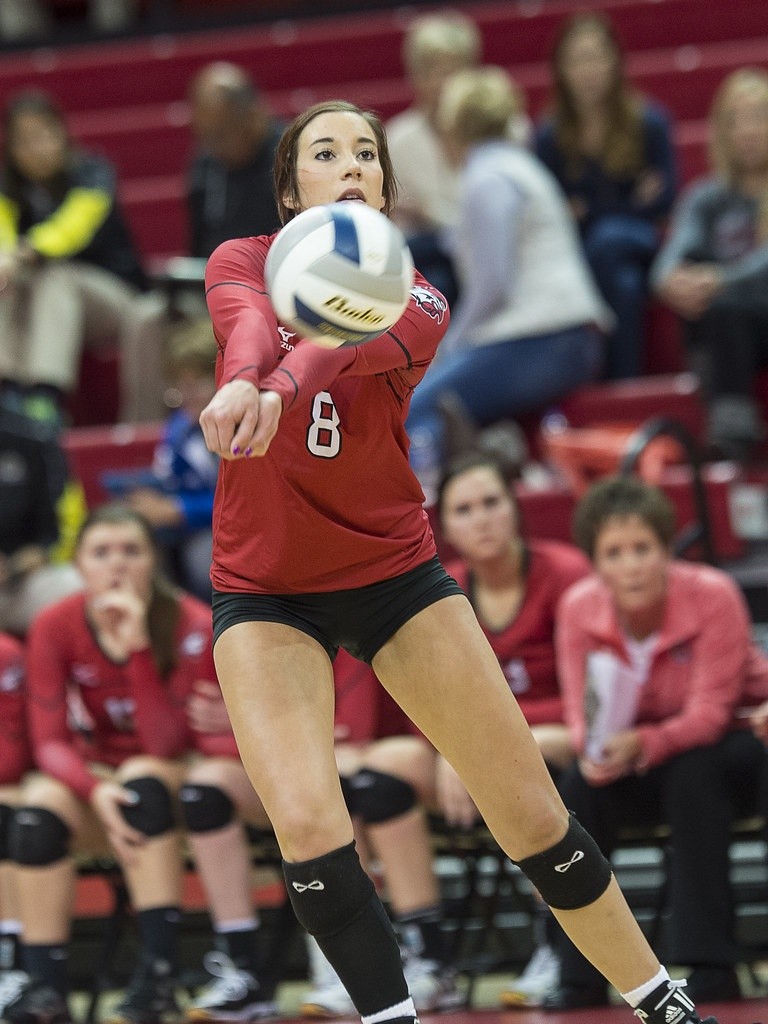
0,0,768,674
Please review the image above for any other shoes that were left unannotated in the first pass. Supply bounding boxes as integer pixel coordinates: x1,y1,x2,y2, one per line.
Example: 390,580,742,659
302,979,356,1016
0,969,31,1017
402,959,468,1012
3,980,72,1024
113,973,189,1023
543,987,610,1013
187,951,278,1022
632,980,720,1023
501,945,563,1006
680,968,742,1003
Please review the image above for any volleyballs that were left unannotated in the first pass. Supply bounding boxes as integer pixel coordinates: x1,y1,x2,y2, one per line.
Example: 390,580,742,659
263,203,412,350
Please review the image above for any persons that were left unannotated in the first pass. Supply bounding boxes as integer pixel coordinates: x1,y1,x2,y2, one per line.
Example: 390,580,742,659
406,67,616,511
200,100,718,1024
0,0,768,1024
